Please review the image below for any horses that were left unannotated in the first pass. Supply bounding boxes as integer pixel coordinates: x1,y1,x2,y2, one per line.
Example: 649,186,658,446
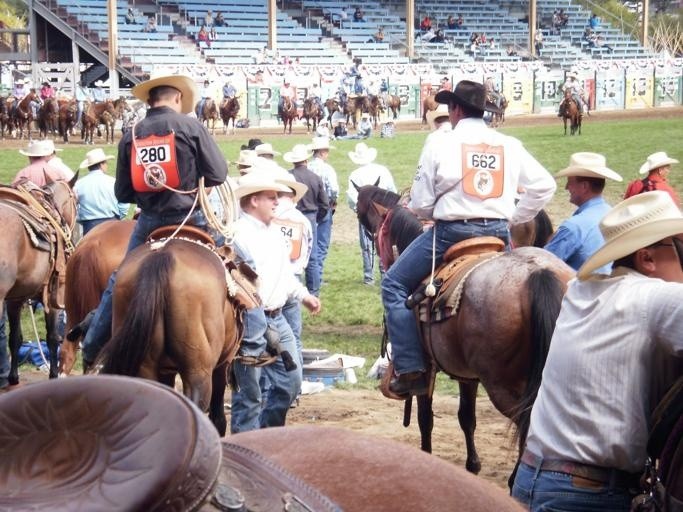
0,94,131,147
484,89,499,128
350,176,555,263
559,89,582,134
0,169,81,390
323,92,401,129
499,91,509,124
194,100,218,137
421,94,440,119
85,233,245,437
370,197,580,479
278,95,297,132
299,97,322,130
195,423,522,511
220,96,239,134
55,218,139,381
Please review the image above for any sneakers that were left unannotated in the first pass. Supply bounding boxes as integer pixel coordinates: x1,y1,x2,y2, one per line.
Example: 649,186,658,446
558,114,562,117
580,113,583,117
240,352,274,367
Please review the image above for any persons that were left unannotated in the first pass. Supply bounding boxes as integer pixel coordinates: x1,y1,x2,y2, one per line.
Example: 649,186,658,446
447,15,456,28
254,45,300,64
253,143,289,175
120,106,133,135
9,79,33,120
80,69,279,367
195,79,220,121
456,14,464,29
207,27,218,41
345,142,397,285
557,72,584,118
354,74,369,96
622,151,680,209
590,13,604,29
230,150,257,176
557,8,568,26
374,27,384,42
582,27,613,52
254,70,265,85
468,31,486,51
40,139,75,183
483,75,500,109
489,37,496,49
84,79,106,104
353,8,365,21
0,301,9,390
124,8,139,24
506,46,517,55
9,139,68,191
337,77,349,111
23,77,31,97
420,26,440,42
306,136,339,290
148,16,156,29
377,77,388,109
435,29,450,43
145,22,157,32
225,170,322,436
36,82,58,118
303,82,325,115
282,144,327,299
551,10,562,29
72,147,130,238
541,152,623,277
198,24,208,40
213,13,229,26
277,78,298,116
438,77,451,92
534,28,544,56
510,188,682,511
420,15,431,32
204,9,214,26
340,6,350,21
269,172,313,407
218,78,240,111
379,79,558,396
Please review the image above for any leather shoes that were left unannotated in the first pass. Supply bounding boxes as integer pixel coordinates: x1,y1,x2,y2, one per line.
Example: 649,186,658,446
389,370,427,395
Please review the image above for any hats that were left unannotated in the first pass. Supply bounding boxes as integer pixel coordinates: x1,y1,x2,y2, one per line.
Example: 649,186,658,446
348,144,377,165
254,144,280,157
39,141,64,152
283,145,314,163
426,104,449,123
577,190,683,283
234,150,258,166
338,119,347,123
435,80,501,113
554,153,622,184
384,119,393,124
131,68,202,114
274,174,308,204
361,114,369,119
80,148,116,172
319,119,329,125
240,138,264,150
240,160,283,173
234,171,293,200
640,151,679,177
568,73,577,79
306,138,336,151
19,140,51,156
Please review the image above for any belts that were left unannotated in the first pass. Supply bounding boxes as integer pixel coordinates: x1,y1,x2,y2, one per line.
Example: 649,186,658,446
264,308,283,319
520,449,638,492
439,218,510,224
